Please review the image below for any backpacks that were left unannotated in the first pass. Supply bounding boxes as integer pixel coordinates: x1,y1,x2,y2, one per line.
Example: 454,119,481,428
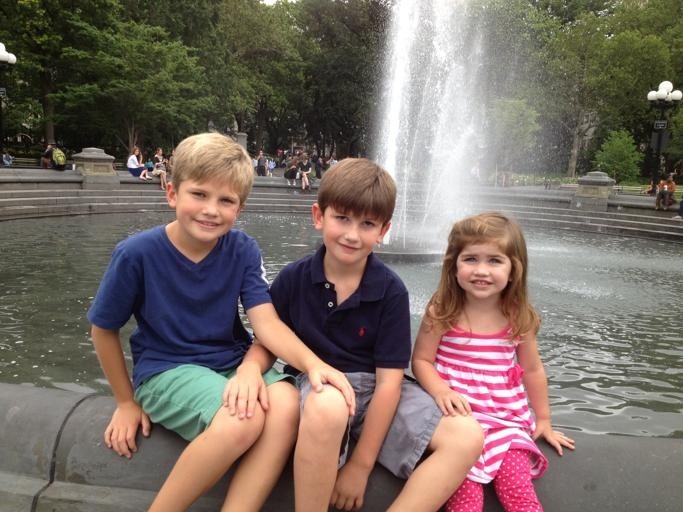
52,148,67,165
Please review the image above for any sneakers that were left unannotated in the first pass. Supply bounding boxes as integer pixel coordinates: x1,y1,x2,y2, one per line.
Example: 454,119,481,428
672,215,681,220
139,176,152,180
302,184,311,194
161,187,166,191
287,180,297,186
655,205,669,210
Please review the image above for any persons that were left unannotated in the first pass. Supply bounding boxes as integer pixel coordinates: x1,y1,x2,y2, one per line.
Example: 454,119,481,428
221,155,486,511
653,174,675,210
2,151,12,168
83,130,357,512
657,179,669,191
642,179,657,196
39,144,65,171
125,146,339,192
411,212,577,512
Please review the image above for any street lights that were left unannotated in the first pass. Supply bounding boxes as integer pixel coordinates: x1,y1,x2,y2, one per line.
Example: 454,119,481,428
647,80,683,196
